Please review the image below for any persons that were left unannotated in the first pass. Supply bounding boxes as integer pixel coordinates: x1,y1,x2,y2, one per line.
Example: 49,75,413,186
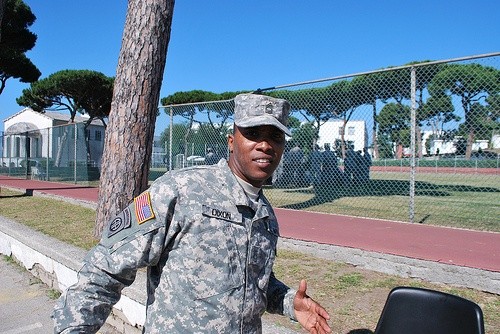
273,142,372,187
204,148,217,165
53,93,331,334
163,149,174,171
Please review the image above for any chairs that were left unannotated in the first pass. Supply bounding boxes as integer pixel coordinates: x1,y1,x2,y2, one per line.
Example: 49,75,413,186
346,285,485,334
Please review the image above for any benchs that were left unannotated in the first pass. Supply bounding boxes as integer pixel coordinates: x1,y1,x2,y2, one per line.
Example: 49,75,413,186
31,166,47,181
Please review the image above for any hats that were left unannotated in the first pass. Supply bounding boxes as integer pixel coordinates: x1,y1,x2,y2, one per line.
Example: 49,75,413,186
234,93,292,137
